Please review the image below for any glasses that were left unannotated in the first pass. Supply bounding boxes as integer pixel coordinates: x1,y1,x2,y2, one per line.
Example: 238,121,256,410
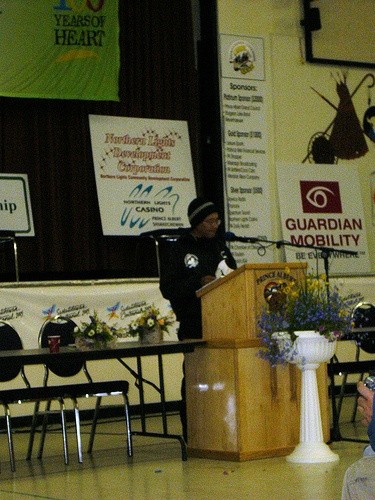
203,219,221,226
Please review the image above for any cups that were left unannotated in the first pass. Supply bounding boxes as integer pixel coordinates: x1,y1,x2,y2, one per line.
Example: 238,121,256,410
48,335,60,353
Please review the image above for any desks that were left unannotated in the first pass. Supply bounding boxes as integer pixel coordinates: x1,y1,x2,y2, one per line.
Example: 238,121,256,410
0,339,205,461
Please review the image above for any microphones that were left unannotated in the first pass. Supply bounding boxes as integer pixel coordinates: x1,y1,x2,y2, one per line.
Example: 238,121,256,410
224,231,248,243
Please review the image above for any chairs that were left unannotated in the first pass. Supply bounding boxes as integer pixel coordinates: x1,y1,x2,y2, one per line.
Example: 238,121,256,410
327,303,375,422
37,315,133,463
0,320,70,471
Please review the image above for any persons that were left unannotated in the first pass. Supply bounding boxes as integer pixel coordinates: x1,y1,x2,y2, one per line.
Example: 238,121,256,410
159,197,236,443
341,381,375,500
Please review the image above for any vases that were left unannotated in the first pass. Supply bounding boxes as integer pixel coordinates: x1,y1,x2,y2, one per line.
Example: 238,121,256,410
271,331,340,463
141,324,161,342
89,337,109,349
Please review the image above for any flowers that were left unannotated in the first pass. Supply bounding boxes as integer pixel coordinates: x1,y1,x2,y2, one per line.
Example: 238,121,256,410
254,264,365,367
73,304,175,341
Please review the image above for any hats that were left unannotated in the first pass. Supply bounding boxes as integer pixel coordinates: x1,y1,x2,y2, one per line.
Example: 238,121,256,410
188,198,216,228
